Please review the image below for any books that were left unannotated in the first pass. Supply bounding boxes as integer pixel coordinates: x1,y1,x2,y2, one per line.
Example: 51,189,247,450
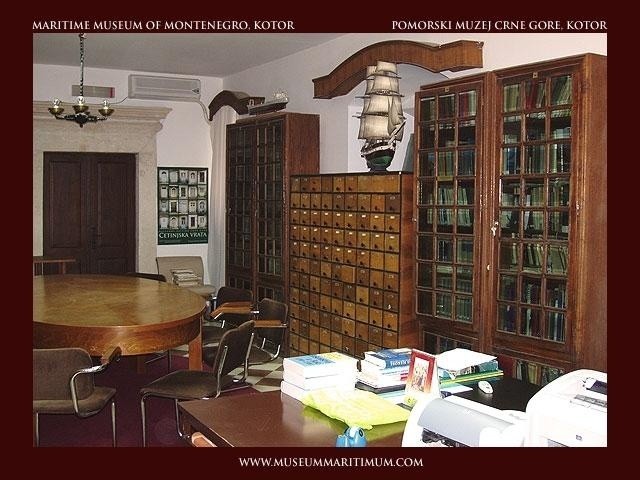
526,81,544,109
551,127,570,140
548,212,568,240
513,360,565,387
500,275,517,301
457,241,474,263
364,347,412,369
439,339,453,354
437,240,452,262
456,299,472,322
355,382,406,395
459,92,476,118
525,181,543,206
503,146,521,175
170,269,199,288
524,244,543,271
438,97,455,119
439,151,454,176
280,380,355,401
420,152,435,175
423,182,432,204
510,243,519,269
521,308,539,337
503,84,521,111
420,209,432,225
457,266,472,293
551,77,571,106
438,128,455,147
437,264,453,290
499,306,517,332
500,211,519,234
544,311,565,342
421,100,435,121
361,360,410,376
546,283,565,309
550,144,570,173
437,348,504,386
458,126,475,145
526,127,546,141
502,134,518,145
502,182,520,206
525,145,545,174
522,278,540,304
548,180,569,206
438,182,453,205
283,352,359,379
547,245,567,275
458,186,474,205
282,369,358,391
437,294,451,318
438,208,453,227
524,210,544,236
457,208,473,226
459,151,475,175
355,369,410,389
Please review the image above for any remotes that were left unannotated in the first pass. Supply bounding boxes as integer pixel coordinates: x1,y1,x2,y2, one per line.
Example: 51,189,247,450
477,381,494,393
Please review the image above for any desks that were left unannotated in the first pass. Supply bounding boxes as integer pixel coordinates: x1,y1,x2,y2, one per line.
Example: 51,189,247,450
33,273,207,371
177,390,406,447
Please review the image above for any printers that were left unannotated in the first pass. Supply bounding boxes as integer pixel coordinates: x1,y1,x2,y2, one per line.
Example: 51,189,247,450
401,394,549,448
525,368,607,447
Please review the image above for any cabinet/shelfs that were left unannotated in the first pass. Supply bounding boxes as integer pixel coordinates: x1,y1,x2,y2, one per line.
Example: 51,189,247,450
415,53,607,387
225,112,320,307
288,171,416,360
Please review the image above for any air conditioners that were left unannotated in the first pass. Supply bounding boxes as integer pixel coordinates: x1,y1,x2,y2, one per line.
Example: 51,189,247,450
128,74,202,102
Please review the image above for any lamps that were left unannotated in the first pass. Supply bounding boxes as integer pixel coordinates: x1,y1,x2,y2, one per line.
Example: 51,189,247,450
47,33,115,128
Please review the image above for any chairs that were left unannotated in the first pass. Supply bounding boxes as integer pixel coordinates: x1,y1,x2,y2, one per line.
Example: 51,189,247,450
156,255,217,319
216,286,254,311
138,320,256,447
33,346,122,447
210,298,288,366
